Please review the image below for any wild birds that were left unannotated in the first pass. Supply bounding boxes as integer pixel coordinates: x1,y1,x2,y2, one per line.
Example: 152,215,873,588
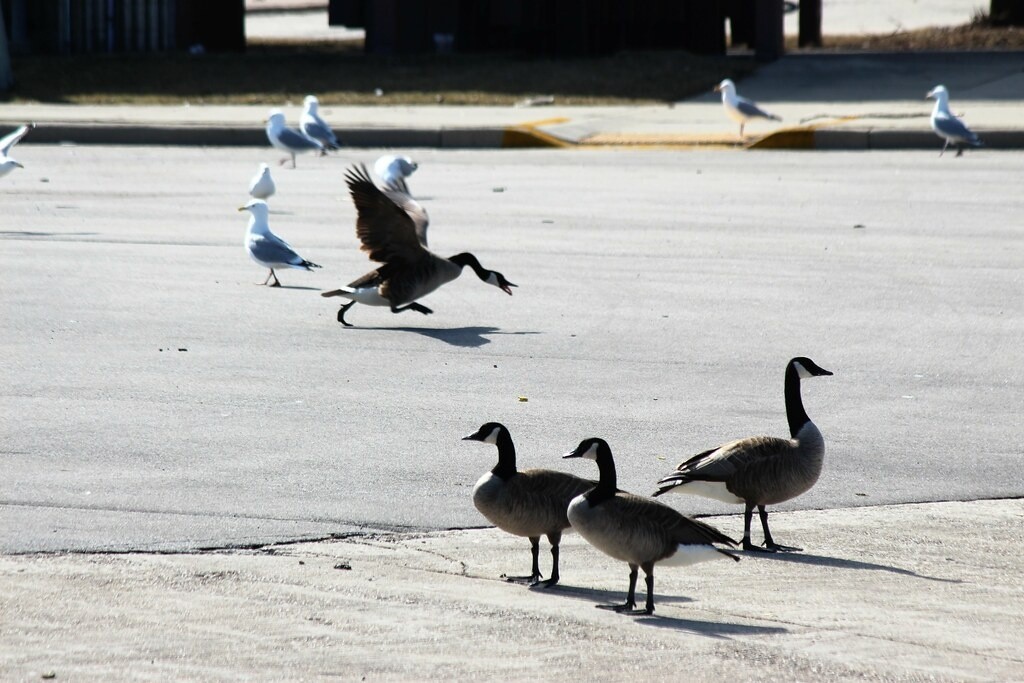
711,78,785,143
0,120,40,181
923,83,985,160
320,152,521,330
459,355,836,617
235,161,330,289
259,94,346,171
